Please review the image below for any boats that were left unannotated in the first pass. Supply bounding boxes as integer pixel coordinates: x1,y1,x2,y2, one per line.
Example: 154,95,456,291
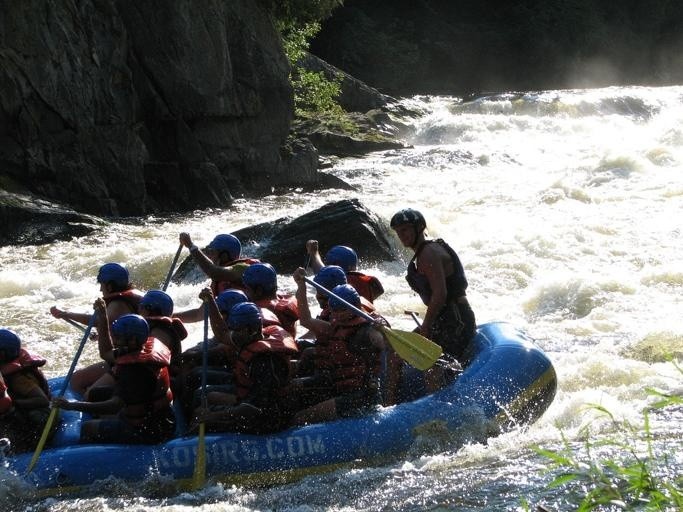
0,319,558,505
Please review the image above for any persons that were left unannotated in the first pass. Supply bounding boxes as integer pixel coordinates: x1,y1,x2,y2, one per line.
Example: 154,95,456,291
171,233,300,433
0,329,51,454
292,240,391,426
49,262,183,445
386,210,476,407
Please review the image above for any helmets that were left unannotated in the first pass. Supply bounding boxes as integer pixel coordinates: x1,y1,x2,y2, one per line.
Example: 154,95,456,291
314,245,361,306
0,329,20,358
97,263,173,339
205,234,277,327
390,208,426,227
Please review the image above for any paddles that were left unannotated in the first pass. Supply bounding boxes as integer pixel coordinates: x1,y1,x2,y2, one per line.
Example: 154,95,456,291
193,294,208,490
22,310,97,472
304,275,441,371
